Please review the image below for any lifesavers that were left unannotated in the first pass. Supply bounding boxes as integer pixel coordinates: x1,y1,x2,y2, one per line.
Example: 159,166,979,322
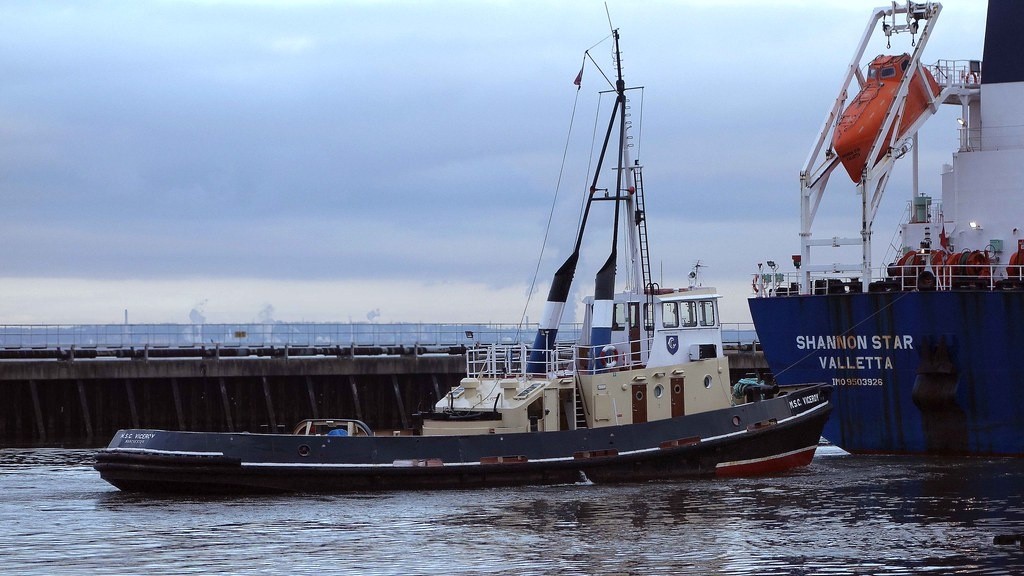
965,72,978,84
600,346,619,368
753,276,759,292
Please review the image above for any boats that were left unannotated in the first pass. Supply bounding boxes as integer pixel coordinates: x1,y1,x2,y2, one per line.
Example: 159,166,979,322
743,0,1024,460
88,0,835,495
830,52,945,185
